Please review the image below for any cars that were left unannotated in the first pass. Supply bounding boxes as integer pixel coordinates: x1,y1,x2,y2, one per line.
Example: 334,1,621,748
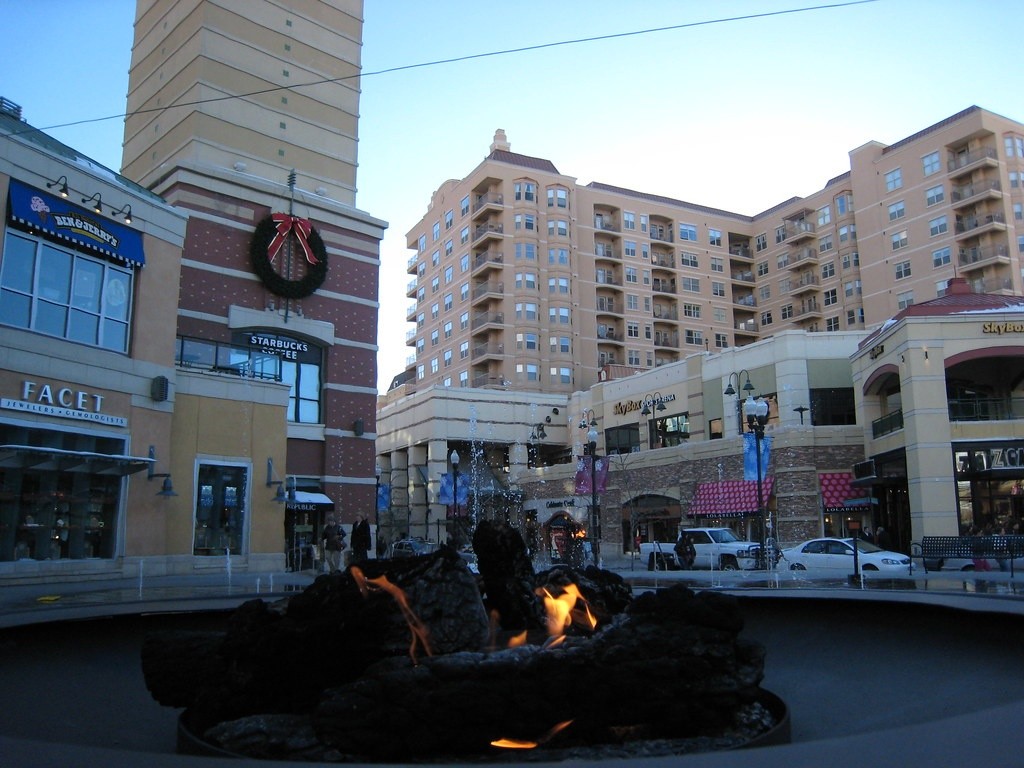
941,554,1024,572
776,536,916,572
386,537,437,559
456,544,478,564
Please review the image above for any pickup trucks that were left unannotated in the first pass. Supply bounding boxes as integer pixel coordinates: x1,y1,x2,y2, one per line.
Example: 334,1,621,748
639,528,776,572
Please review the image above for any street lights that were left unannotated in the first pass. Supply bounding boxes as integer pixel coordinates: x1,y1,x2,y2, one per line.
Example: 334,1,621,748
745,397,770,570
578,426,599,571
375,463,381,557
451,447,459,539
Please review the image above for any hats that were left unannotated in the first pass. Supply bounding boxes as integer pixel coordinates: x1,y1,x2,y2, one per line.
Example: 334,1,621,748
327,515,335,521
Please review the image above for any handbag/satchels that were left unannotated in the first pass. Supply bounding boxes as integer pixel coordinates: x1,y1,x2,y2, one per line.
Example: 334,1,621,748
337,540,347,552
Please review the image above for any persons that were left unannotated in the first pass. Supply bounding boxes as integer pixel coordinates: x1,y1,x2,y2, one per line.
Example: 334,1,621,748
322,514,346,570
862,527,892,550
969,521,1024,570
350,511,372,560
675,532,695,569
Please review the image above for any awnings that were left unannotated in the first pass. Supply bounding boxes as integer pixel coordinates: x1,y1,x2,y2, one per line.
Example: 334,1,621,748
819,474,868,511
688,477,774,518
0,444,156,478
287,492,335,512
